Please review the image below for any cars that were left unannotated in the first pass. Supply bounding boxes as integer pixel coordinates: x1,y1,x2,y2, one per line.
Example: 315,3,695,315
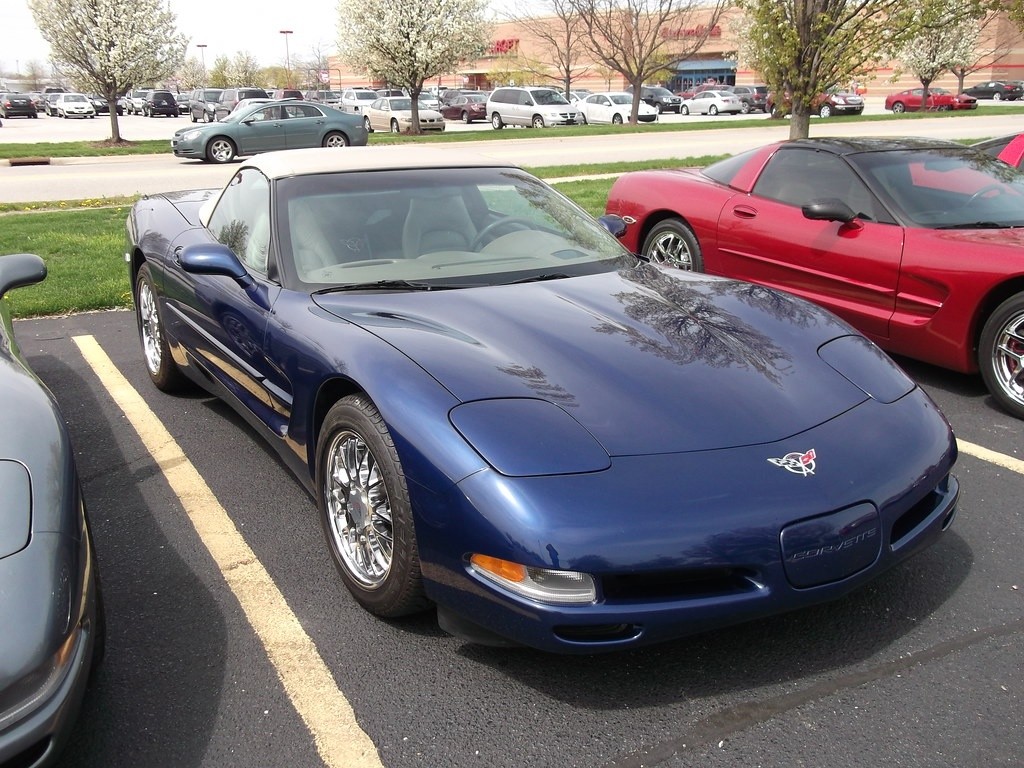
370,96,446,135
439,94,488,125
264,87,370,108
909,132,1024,200
765,83,864,118
884,87,978,115
0,86,190,117
576,91,659,123
533,84,594,106
679,90,742,115
419,85,487,111
169,97,368,163
375,89,404,98
963,80,1024,101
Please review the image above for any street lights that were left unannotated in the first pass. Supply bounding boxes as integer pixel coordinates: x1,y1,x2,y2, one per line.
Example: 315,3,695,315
196,44,209,91
280,30,294,89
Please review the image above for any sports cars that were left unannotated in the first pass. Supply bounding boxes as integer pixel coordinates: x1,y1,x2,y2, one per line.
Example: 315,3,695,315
603,135,1024,423
1,255,105,768
124,145,962,654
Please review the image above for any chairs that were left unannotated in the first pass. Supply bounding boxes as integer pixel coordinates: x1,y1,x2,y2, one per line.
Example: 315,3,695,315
254,200,335,272
401,197,481,258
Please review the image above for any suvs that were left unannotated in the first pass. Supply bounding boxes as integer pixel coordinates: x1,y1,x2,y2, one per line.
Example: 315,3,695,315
190,87,224,124
213,87,270,122
339,90,385,121
486,85,584,130
728,84,770,114
621,84,683,114
677,83,731,102
